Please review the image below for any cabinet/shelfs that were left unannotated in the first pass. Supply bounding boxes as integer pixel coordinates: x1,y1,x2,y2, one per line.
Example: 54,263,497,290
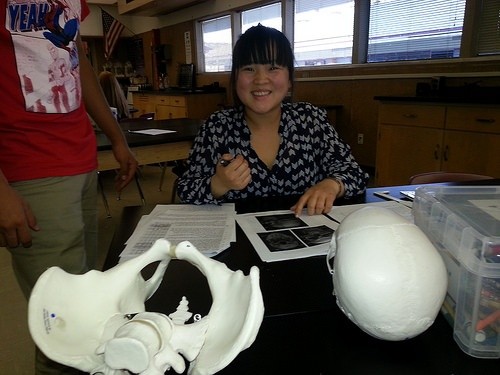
132,90,227,121
374,96,500,186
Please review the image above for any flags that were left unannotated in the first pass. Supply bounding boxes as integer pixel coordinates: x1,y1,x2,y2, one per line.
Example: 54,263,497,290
98,8,125,60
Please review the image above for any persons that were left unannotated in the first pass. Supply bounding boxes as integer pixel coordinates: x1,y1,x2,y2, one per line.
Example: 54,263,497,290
171,23,369,218
0,0,138,375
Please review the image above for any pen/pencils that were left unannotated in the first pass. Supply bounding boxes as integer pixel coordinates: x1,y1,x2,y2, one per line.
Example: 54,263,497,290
221,159,229,165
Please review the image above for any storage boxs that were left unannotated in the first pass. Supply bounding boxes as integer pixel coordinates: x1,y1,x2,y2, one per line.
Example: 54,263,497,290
412,185,500,359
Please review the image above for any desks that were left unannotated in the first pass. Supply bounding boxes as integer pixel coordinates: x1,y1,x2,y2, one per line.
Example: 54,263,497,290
90,192,500,375
95,121,198,214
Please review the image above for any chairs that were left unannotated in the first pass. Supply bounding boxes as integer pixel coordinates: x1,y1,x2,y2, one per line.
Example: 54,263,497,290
99,70,155,119
